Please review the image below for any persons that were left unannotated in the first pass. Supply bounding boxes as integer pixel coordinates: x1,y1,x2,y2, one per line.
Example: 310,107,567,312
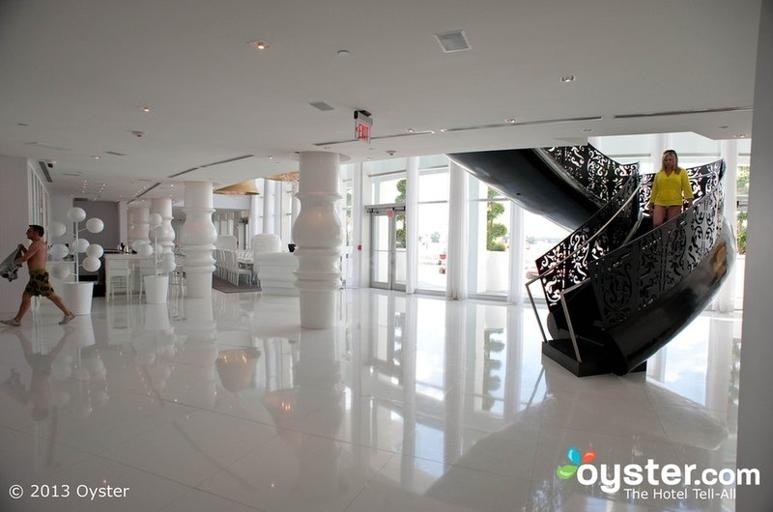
646,149,693,231
0,223,75,326
0,328,75,423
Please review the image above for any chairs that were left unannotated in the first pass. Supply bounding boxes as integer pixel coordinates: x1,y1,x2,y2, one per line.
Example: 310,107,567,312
107,259,186,302
214,248,252,288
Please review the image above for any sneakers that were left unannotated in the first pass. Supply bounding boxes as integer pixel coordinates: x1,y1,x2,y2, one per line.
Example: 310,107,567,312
0,318,21,327
58,312,76,325
